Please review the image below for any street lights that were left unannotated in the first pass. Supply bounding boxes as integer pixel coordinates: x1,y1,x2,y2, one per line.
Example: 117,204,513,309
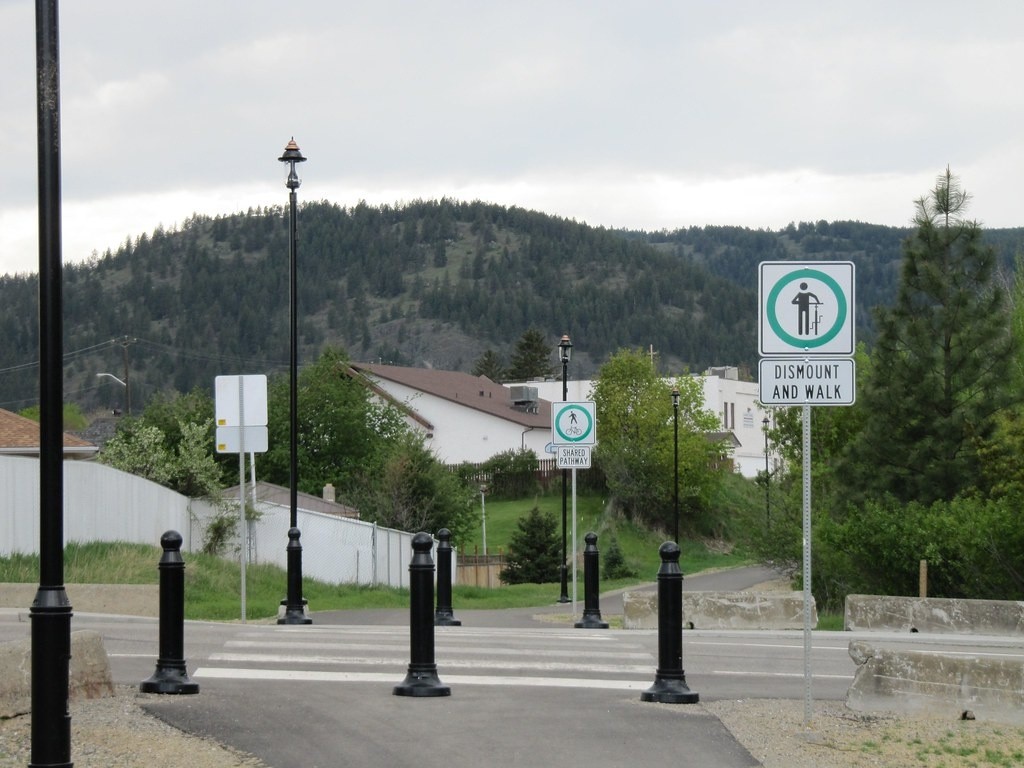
480,484,488,555
668,385,682,544
92,370,131,415
557,334,575,603
272,134,315,627
